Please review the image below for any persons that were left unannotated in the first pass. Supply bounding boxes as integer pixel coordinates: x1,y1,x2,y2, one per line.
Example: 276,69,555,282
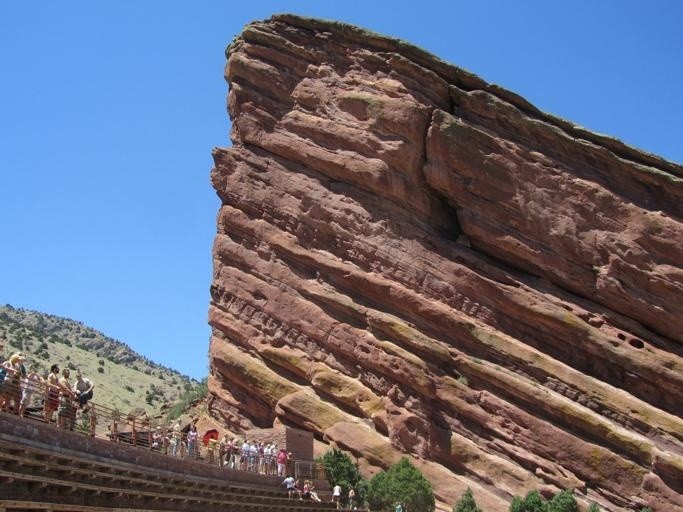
394,502,402,512
0,352,95,431
346,486,355,511
330,483,342,510
148,418,322,505
